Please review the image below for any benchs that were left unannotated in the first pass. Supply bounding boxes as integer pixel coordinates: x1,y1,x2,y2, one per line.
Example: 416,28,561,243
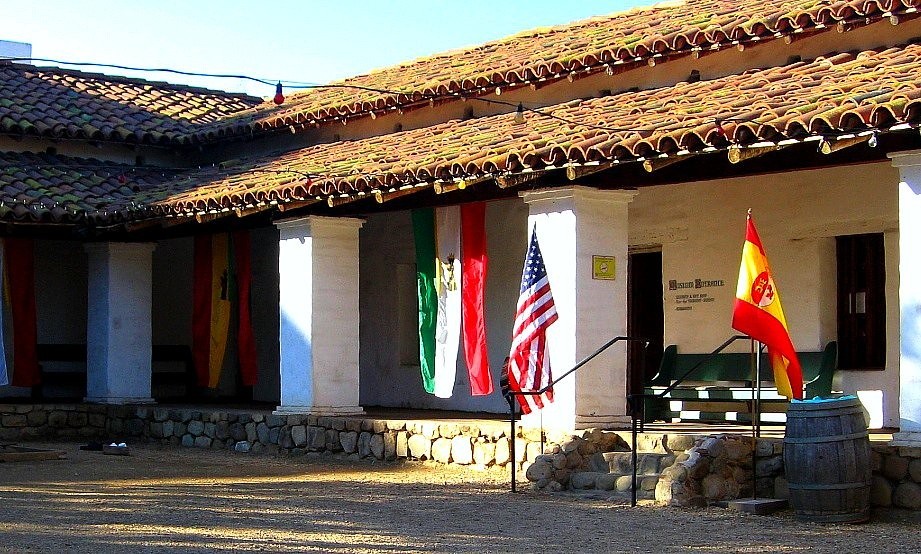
31,344,195,400
641,339,838,428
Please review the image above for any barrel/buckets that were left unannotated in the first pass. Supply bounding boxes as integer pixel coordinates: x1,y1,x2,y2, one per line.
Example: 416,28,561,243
783,395,871,523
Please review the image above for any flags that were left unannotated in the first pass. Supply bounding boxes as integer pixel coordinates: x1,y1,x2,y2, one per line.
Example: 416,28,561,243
411,203,494,399
1,232,41,387
506,222,559,415
731,212,803,401
192,230,259,389
